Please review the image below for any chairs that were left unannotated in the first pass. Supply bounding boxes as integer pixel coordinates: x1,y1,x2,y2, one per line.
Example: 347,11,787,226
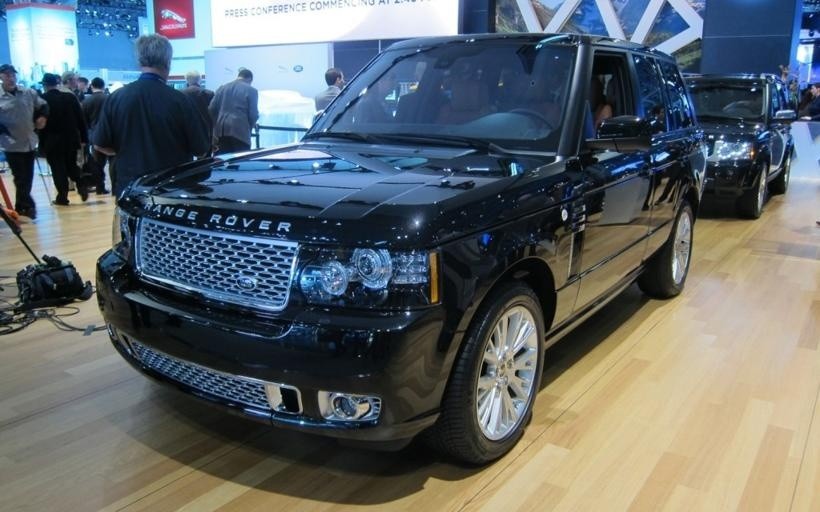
434,80,490,125
512,72,545,107
605,79,622,116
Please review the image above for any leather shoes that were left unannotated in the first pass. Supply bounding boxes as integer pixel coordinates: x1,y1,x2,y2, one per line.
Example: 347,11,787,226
51,187,109,206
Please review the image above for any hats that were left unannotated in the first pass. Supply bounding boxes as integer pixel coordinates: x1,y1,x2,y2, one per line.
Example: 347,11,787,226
0,64,18,74
38,73,57,85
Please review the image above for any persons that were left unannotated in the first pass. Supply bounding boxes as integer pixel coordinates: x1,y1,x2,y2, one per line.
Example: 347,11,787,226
640,171,661,211
779,63,820,123
353,71,400,123
0,63,111,220
589,75,613,132
92,33,214,248
315,67,347,111
656,170,678,205
207,68,260,155
178,67,215,159
